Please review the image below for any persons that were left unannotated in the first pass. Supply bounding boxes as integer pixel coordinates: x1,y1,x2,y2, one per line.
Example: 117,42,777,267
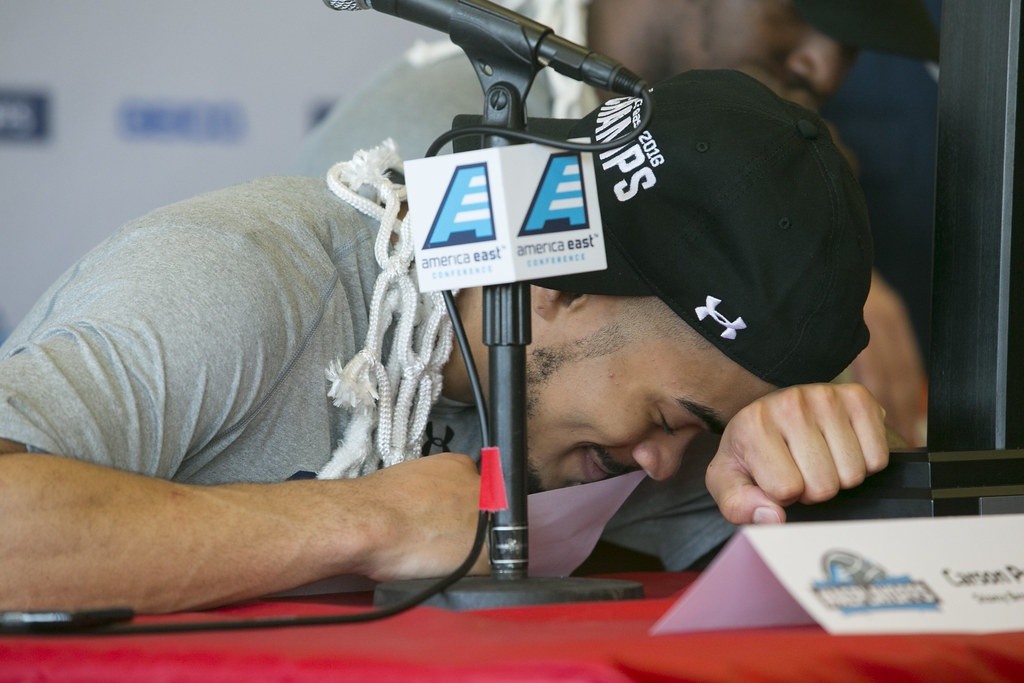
0,70,890,613
279,0,930,448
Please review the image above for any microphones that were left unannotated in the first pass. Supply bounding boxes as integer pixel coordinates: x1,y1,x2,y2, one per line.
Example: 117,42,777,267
323,0,649,98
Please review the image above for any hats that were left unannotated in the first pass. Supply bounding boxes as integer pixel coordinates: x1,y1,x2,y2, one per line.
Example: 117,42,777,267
451,69,871,389
792,0,942,64
